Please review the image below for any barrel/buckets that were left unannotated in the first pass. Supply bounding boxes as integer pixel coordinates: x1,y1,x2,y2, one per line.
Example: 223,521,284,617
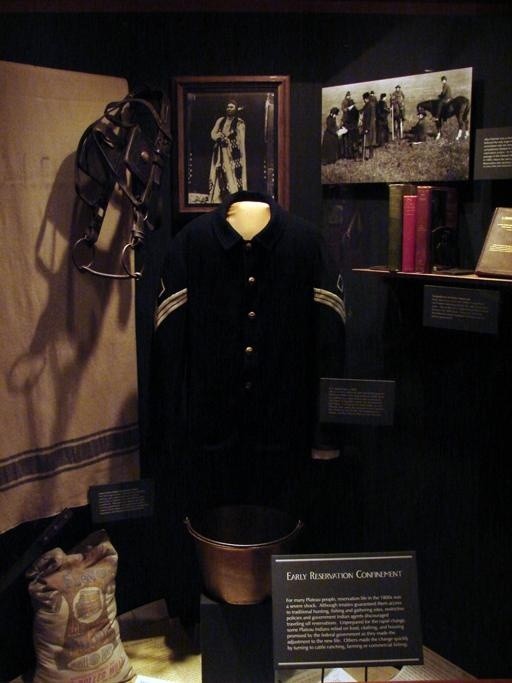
183,506,304,606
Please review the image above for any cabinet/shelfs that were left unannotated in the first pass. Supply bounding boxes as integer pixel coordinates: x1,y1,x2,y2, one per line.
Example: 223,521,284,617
344,266,512,682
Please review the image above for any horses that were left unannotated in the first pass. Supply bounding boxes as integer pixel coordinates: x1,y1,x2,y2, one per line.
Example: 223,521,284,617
416,94,470,141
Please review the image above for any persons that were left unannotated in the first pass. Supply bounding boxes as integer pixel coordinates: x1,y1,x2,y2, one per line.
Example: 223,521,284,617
147,195,346,553
321,85,406,166
413,111,431,148
436,77,454,123
210,96,247,206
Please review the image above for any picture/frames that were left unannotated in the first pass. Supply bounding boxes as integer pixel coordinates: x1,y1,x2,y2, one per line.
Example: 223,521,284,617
319,63,474,185
176,74,293,219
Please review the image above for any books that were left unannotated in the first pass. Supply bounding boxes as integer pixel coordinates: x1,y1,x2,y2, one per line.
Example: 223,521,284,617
474,207,512,280
385,182,418,273
401,195,418,273
416,185,460,273
